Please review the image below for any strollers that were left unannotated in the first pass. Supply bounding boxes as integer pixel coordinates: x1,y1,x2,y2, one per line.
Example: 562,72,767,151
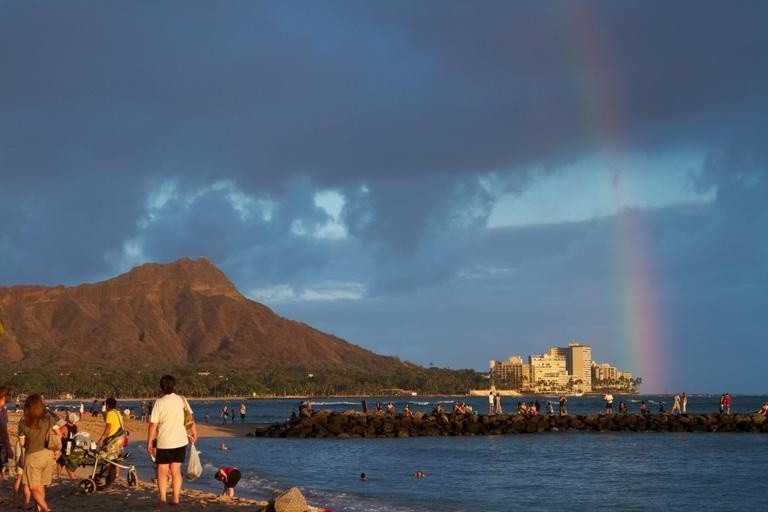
60,427,137,496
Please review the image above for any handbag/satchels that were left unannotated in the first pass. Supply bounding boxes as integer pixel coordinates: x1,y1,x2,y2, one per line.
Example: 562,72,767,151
45,412,63,452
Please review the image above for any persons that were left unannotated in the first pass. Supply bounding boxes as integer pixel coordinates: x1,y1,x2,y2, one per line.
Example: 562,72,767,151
213,465,243,498
361,391,570,416
286,398,315,421
639,401,651,416
618,401,628,416
671,393,681,415
758,401,768,416
723,393,732,416
151,439,172,489
0,385,156,512
239,401,248,425
220,405,230,424
230,407,237,424
220,435,231,453
603,390,615,414
681,391,690,415
658,399,666,414
719,392,726,415
146,374,199,509
414,470,427,477
359,471,369,482
